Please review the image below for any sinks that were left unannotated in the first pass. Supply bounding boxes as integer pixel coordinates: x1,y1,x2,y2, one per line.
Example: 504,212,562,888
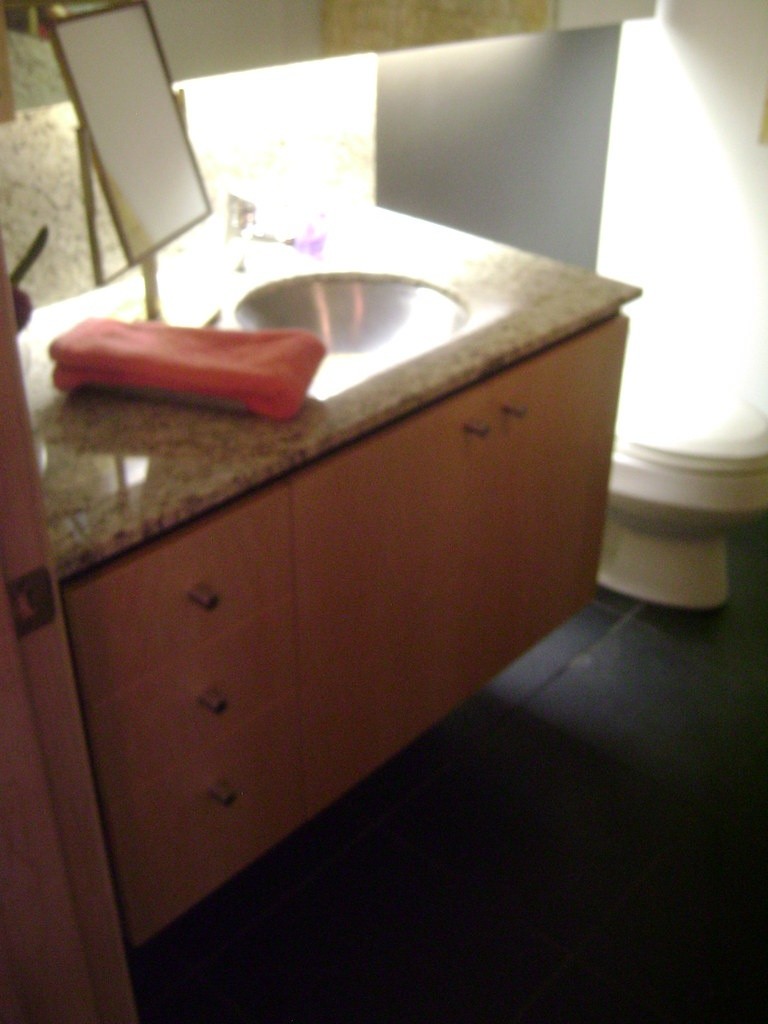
233,269,475,361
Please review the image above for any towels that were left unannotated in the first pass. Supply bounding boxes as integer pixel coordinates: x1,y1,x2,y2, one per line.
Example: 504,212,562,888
49,315,325,420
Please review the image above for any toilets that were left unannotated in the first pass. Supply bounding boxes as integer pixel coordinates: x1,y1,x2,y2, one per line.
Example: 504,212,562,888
597,369,768,608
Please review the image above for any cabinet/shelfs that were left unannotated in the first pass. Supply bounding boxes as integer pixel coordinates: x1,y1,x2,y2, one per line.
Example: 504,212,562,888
60,308,628,949
